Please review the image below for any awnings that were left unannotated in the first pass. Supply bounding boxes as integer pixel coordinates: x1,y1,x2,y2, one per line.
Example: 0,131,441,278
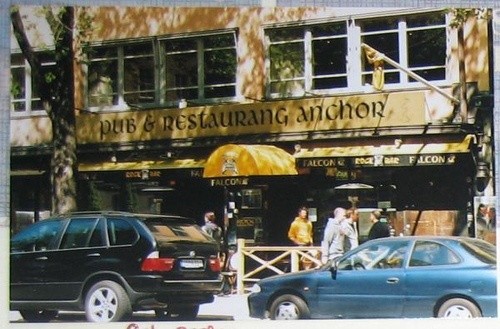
11,163,47,184
79,137,476,179
203,145,311,177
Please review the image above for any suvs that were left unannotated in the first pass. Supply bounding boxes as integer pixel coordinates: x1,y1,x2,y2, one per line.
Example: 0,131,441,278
9,210,224,322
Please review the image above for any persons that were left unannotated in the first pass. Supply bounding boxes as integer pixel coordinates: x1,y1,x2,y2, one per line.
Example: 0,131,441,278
341,207,359,250
201,212,218,234
477,204,487,228
368,210,391,251
488,208,496,229
288,206,313,246
321,207,350,265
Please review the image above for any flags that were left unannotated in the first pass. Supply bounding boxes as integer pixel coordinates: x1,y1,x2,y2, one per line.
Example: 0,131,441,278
366,49,384,90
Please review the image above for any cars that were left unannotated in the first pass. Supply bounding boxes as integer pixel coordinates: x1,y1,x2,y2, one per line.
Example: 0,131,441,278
247,235,497,319
